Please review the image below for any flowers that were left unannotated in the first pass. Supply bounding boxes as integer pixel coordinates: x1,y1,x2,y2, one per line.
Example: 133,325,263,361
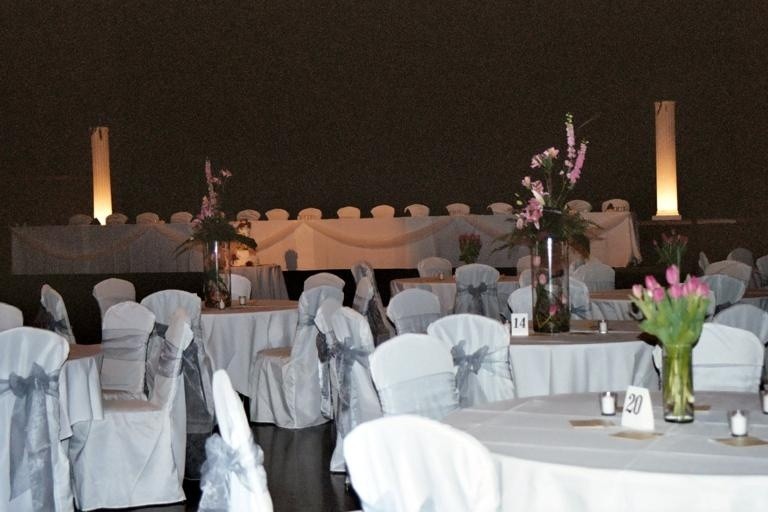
651,229,687,263
491,112,591,333
627,264,710,416
457,232,483,264
168,157,258,308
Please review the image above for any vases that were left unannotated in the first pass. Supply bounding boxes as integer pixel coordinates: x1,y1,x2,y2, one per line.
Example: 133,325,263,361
530,237,570,333
201,236,233,313
662,344,693,423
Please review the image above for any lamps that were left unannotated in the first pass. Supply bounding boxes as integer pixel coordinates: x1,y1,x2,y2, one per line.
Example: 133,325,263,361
653,101,682,222
89,125,114,222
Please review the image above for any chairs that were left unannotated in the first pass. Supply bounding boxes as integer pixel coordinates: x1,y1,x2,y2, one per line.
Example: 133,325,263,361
698,251,709,269
573,260,616,289
696,275,747,305
370,205,395,219
35,284,74,343
170,211,191,223
338,206,359,218
564,198,592,212
369,333,458,416
250,286,344,429
344,414,499,512
426,314,515,408
385,289,439,333
600,199,630,213
517,254,530,272
756,255,767,284
418,257,452,277
506,278,602,318
298,206,321,220
651,323,765,391
105,213,127,224
68,215,91,227
265,206,289,219
704,261,752,277
350,261,390,334
199,369,275,512
727,248,755,268
0,326,71,512
404,203,429,216
234,207,260,220
140,289,214,434
230,273,252,299
520,268,532,286
74,307,197,512
100,301,157,401
352,277,374,314
92,277,136,318
447,204,472,216
455,263,501,315
303,273,345,290
488,203,513,214
714,304,768,345
135,211,159,222
0,302,24,326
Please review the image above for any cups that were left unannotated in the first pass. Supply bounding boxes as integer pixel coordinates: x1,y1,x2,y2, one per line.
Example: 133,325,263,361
238,295,246,305
726,408,749,437
598,391,618,416
598,319,608,335
758,389,767,414
438,272,444,280
